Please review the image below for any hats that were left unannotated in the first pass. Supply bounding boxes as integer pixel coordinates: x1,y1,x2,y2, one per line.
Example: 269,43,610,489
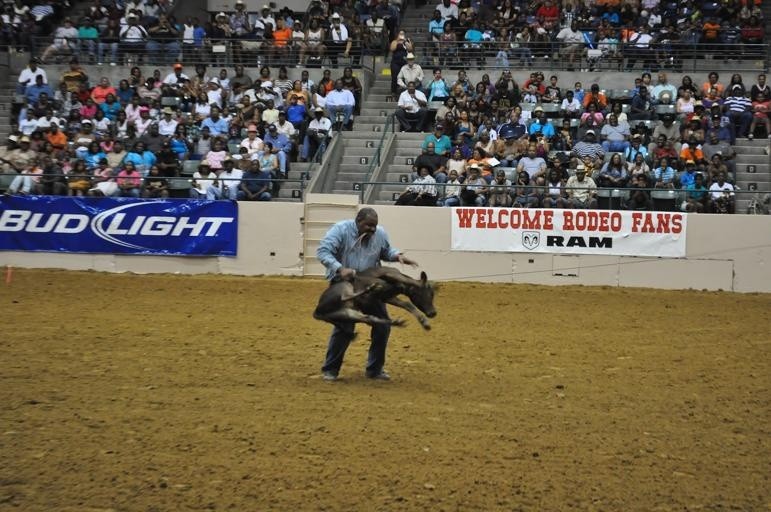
268,124,276,131
403,52,417,60
694,101,704,107
534,106,543,113
126,13,139,22
659,90,673,104
328,13,344,23
20,136,32,143
8,135,17,143
219,154,239,167
209,77,219,86
174,64,183,69
140,106,150,111
200,160,212,166
215,12,229,22
467,163,483,175
585,129,596,136
261,5,271,12
691,115,701,122
315,107,323,112
573,165,588,174
248,125,257,132
161,107,175,114
234,0,246,10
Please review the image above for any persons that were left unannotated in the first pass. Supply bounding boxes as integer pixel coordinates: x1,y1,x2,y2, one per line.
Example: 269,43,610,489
2,2,770,217
316,209,419,382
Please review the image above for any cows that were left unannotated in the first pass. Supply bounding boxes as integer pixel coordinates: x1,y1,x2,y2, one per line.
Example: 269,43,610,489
312,267,442,339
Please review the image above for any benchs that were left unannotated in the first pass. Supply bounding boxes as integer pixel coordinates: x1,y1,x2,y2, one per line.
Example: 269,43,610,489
0,1,771,216
